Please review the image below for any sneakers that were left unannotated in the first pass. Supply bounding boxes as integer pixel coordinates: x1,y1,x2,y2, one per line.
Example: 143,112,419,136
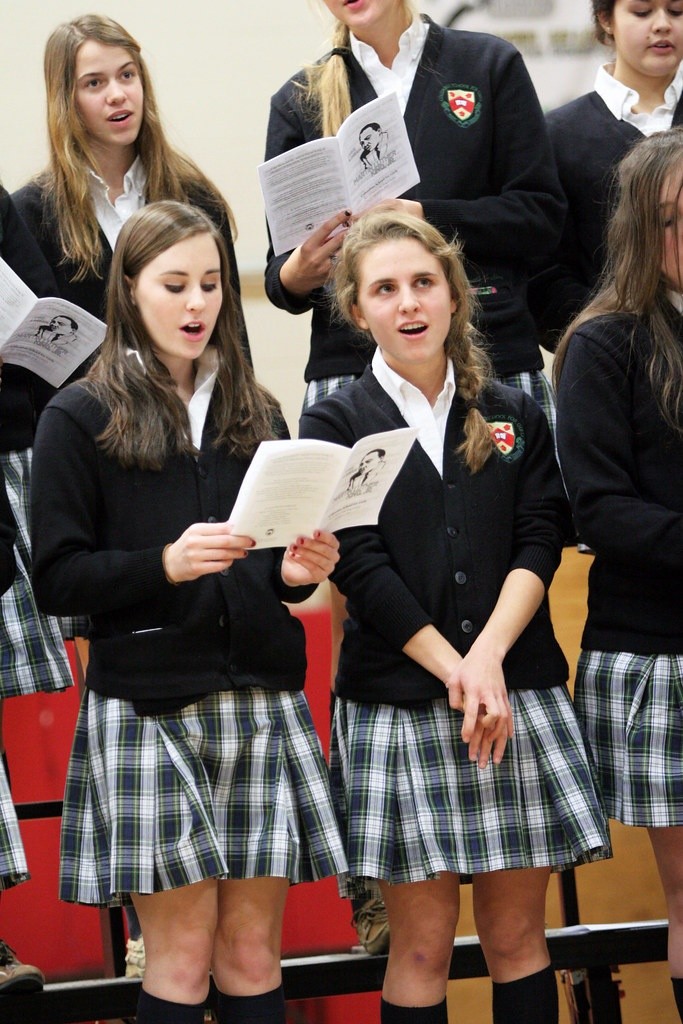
353,897,390,955
1,939,44,994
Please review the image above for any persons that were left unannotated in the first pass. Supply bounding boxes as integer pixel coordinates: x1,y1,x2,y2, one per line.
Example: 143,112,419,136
1,183,95,699
0,470,48,1011
263,0,561,469
31,200,341,1023
13,15,252,395
296,206,615,1024
551,129,683,1022
528,0,683,353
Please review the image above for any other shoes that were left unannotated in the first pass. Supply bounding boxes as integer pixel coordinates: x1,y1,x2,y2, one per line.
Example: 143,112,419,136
124,935,147,979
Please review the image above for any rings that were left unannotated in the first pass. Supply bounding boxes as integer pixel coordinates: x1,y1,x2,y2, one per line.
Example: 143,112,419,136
330,255,339,266
